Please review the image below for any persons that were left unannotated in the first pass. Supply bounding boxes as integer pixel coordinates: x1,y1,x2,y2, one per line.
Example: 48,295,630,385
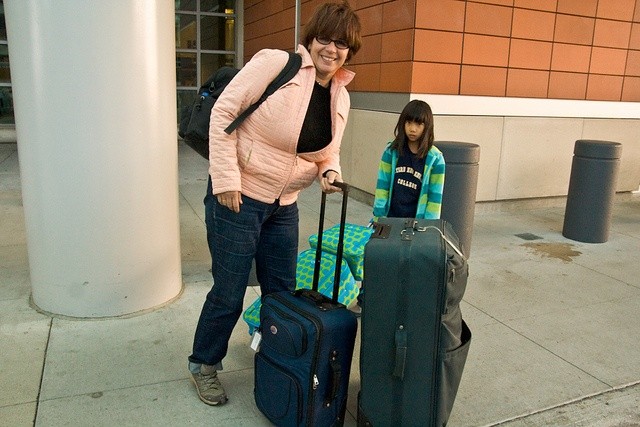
370,98,446,229
178,0,365,410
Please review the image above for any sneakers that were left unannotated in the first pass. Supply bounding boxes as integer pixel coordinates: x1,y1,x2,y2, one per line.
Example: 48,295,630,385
188,364,229,408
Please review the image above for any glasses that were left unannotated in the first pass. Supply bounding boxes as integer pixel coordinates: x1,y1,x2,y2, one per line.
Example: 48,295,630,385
310,31,353,52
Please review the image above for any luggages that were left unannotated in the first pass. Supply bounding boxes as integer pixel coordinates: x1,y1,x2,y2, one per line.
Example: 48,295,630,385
242,249,360,346
356,216,473,427
252,179,357,427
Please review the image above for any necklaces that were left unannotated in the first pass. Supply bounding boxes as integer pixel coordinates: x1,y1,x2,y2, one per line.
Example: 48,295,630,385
315,79,325,88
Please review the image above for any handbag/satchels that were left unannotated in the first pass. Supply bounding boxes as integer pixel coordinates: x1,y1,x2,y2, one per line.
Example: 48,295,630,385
178,48,303,162
308,223,375,281
